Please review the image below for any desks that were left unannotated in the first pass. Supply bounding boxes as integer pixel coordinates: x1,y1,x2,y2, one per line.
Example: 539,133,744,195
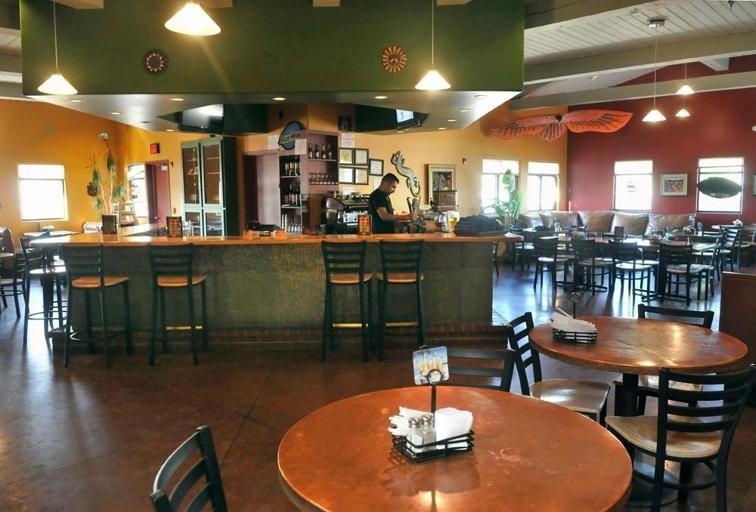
277,385,633,512
528,316,749,466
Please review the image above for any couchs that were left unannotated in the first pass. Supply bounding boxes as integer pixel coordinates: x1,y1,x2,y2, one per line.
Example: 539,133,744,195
518,210,696,238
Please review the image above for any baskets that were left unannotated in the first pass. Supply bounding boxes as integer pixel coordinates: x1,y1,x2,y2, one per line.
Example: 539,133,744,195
552,324,597,345
391,427,475,462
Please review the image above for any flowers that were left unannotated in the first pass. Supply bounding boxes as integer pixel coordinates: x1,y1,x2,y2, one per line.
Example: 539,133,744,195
87,132,125,214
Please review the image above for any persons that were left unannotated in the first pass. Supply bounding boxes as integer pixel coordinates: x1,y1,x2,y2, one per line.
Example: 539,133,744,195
368,173,416,233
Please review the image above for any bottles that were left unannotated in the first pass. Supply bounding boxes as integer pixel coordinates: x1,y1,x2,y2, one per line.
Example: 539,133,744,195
407,415,437,457
308,143,312,159
285,159,300,176
331,190,341,200
411,198,418,217
308,172,338,185
327,144,333,159
321,144,326,159
315,144,320,159
281,180,301,233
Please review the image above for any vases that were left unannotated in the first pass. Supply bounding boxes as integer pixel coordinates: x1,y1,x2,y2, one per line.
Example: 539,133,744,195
101,215,118,241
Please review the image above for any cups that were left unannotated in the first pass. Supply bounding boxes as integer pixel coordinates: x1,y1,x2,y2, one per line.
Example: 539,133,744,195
319,223,327,233
420,208,459,231
182,226,195,235
553,222,560,232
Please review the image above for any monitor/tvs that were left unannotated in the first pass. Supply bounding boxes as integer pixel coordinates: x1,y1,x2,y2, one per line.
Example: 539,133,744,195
177,104,226,135
394,109,421,130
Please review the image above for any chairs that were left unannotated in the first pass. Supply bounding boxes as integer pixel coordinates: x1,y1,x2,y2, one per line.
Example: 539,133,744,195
149,424,229,511
507,312,611,433
605,363,756,511
146,242,207,367
419,344,517,391
0,221,102,354
58,243,130,367
321,239,431,362
613,304,714,416
516,220,756,307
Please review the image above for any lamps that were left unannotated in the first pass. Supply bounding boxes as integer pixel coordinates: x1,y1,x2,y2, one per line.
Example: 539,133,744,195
676,63,694,96
641,18,666,123
675,97,690,118
415,1,451,91
164,1,221,36
37,1,77,95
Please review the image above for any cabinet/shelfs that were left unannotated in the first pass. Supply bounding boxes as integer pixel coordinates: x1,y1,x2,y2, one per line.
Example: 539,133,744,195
182,142,224,236
307,131,341,195
278,154,303,233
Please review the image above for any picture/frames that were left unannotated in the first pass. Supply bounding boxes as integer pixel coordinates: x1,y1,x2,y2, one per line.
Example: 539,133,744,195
659,171,688,197
339,147,384,185
427,163,456,204
